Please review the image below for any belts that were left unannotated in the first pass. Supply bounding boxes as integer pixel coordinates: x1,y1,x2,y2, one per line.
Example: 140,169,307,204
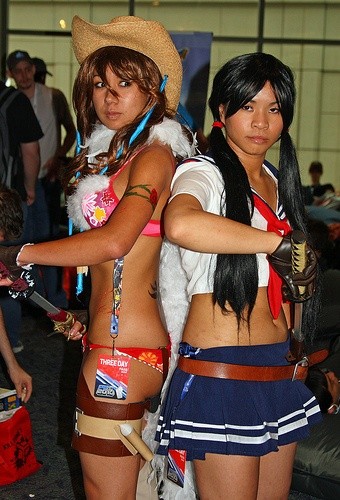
178,347,329,382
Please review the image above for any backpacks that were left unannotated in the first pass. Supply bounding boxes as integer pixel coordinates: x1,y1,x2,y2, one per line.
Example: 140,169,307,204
0,85,21,188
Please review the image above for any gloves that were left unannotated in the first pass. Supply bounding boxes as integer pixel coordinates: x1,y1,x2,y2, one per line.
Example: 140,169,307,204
266,236,321,304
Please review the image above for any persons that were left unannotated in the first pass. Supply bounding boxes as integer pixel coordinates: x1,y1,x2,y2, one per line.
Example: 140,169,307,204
164,53,325,500
0,15,201,500
304,365,340,415
1,51,78,402
301,159,336,210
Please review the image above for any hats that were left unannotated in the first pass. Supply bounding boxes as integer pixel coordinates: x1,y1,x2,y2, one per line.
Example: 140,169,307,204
309,161,323,175
7,50,32,70
71,15,183,119
31,56,54,78
